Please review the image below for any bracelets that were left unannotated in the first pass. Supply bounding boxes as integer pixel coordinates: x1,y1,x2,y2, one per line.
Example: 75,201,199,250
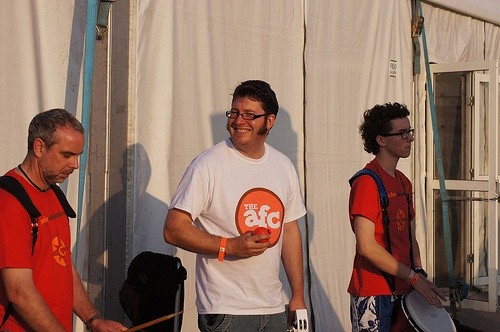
409,274,419,285
218,237,227,261
416,267,428,279
406,269,414,282
85,315,101,328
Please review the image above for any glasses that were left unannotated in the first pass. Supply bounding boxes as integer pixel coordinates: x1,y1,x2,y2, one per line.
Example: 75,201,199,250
226,110,272,121
380,129,417,140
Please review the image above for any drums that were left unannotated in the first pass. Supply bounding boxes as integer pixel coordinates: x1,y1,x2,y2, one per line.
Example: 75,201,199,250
392,289,457,332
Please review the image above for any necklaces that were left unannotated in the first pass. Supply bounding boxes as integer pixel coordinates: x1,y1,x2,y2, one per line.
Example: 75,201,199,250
18,164,50,193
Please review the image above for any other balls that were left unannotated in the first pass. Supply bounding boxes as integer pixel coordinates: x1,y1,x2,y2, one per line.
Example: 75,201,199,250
253,227,271,243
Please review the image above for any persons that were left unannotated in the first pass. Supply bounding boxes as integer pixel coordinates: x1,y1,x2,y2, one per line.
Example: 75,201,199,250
163,80,307,332
347,102,447,332
0,108,128,332
